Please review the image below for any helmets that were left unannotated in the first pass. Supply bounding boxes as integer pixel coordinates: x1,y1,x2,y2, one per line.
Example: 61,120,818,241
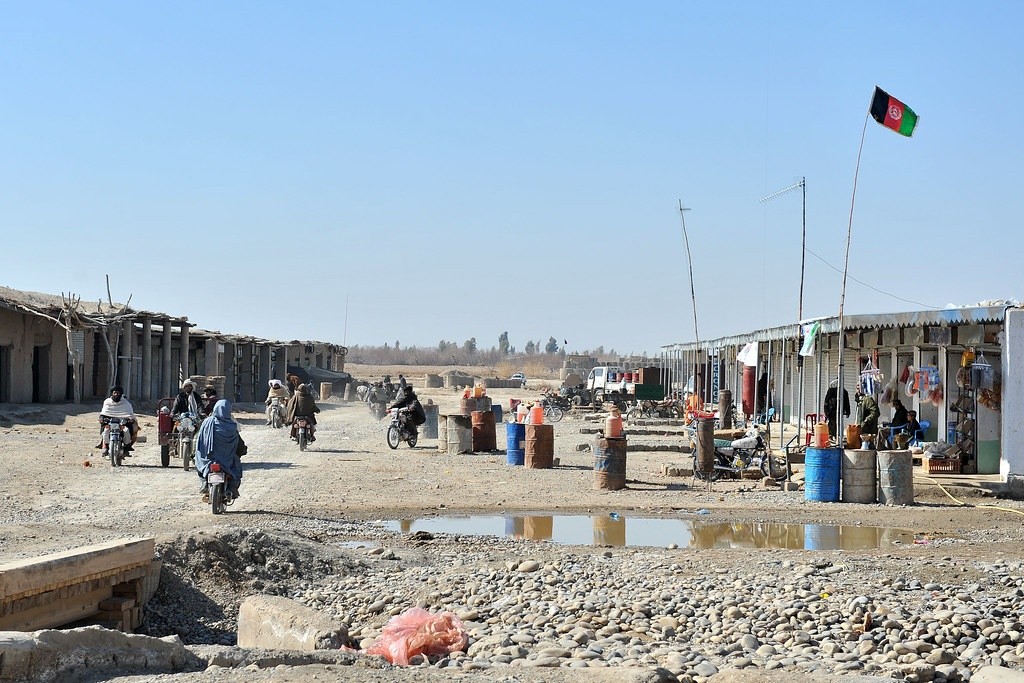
110,385,124,395
272,379,282,388
374,382,378,386
269,380,273,387
402,385,413,397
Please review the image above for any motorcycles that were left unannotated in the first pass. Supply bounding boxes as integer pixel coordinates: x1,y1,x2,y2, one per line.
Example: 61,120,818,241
290,409,320,451
369,400,390,420
681,407,791,482
386,408,420,449
100,418,135,467
509,394,563,422
189,446,247,514
265,395,286,428
157,398,220,471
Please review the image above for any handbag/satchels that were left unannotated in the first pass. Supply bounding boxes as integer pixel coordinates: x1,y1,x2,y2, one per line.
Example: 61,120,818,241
412,400,426,425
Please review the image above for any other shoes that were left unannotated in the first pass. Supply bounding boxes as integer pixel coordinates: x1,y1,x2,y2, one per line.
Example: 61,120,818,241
310,435,316,441
290,434,296,438
95,441,102,449
123,451,129,457
266,420,272,425
123,445,135,451
226,486,240,498
102,449,108,457
199,481,208,493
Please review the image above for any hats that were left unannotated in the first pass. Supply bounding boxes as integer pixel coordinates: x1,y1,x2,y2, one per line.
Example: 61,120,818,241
298,383,307,392
182,379,194,388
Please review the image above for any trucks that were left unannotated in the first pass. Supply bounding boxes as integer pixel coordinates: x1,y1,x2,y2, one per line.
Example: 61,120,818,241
586,366,664,403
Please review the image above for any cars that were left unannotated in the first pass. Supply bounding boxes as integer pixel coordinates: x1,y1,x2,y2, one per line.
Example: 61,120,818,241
511,374,526,386
684,376,694,397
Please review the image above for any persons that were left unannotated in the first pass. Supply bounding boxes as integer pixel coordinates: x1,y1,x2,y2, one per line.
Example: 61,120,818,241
896,410,925,450
685,391,703,424
364,380,390,415
855,380,865,406
286,383,320,440
854,395,880,434
265,379,291,426
399,374,407,389
388,385,426,438
95,386,138,451
290,376,302,391
194,399,248,496
874,399,910,451
384,375,391,384
204,384,220,415
823,376,851,439
169,378,206,456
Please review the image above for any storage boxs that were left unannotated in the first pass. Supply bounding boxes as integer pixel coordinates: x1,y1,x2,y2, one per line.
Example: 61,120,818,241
922,442,962,474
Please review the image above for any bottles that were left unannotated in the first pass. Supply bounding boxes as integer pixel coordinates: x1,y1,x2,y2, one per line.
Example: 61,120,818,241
531,401,542,424
464,385,470,398
815,423,828,449
606,406,622,438
474,383,482,398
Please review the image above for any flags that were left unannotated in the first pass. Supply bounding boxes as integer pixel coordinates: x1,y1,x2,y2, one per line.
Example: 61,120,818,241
870,85,919,138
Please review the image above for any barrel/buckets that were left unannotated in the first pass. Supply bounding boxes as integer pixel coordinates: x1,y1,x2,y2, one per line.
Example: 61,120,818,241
419,405,439,438
876,450,913,505
460,398,492,454
320,382,332,401
805,447,841,502
507,422,553,470
594,438,627,489
471,411,496,452
842,450,877,504
438,413,472,456
491,405,502,423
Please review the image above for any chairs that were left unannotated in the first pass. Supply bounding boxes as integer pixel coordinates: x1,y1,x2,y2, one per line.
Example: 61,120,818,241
805,413,825,446
890,420,931,448
751,408,774,424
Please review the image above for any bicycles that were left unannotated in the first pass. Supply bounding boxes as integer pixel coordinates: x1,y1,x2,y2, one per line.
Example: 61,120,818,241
626,399,680,421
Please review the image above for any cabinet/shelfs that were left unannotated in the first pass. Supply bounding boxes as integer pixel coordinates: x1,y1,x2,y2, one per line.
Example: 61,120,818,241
957,367,976,473
